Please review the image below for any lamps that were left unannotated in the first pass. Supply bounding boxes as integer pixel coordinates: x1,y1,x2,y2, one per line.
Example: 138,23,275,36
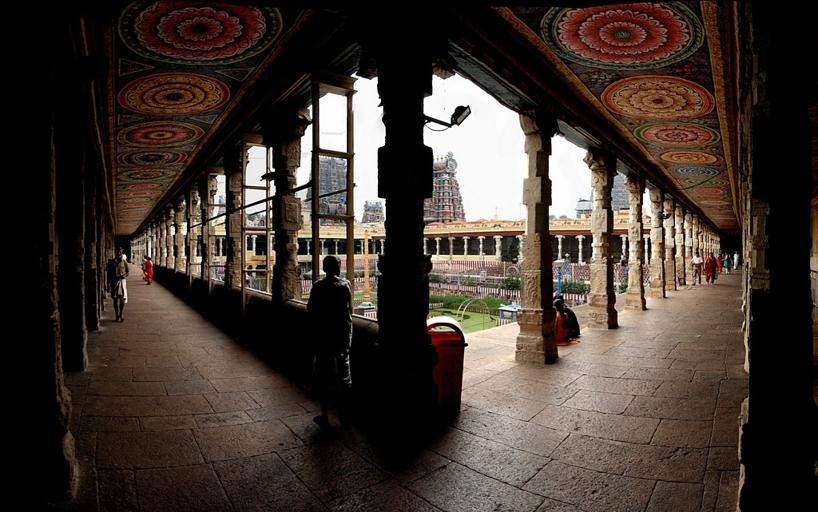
423,100,470,131
663,213,671,220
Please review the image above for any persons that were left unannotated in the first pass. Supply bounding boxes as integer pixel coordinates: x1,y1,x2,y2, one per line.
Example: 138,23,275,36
553,298,579,347
304,255,354,433
106,246,130,322
555,294,580,340
688,250,740,287
141,255,154,285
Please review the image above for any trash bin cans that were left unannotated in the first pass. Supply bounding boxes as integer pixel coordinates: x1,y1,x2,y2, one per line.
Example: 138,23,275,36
427,315,468,413
